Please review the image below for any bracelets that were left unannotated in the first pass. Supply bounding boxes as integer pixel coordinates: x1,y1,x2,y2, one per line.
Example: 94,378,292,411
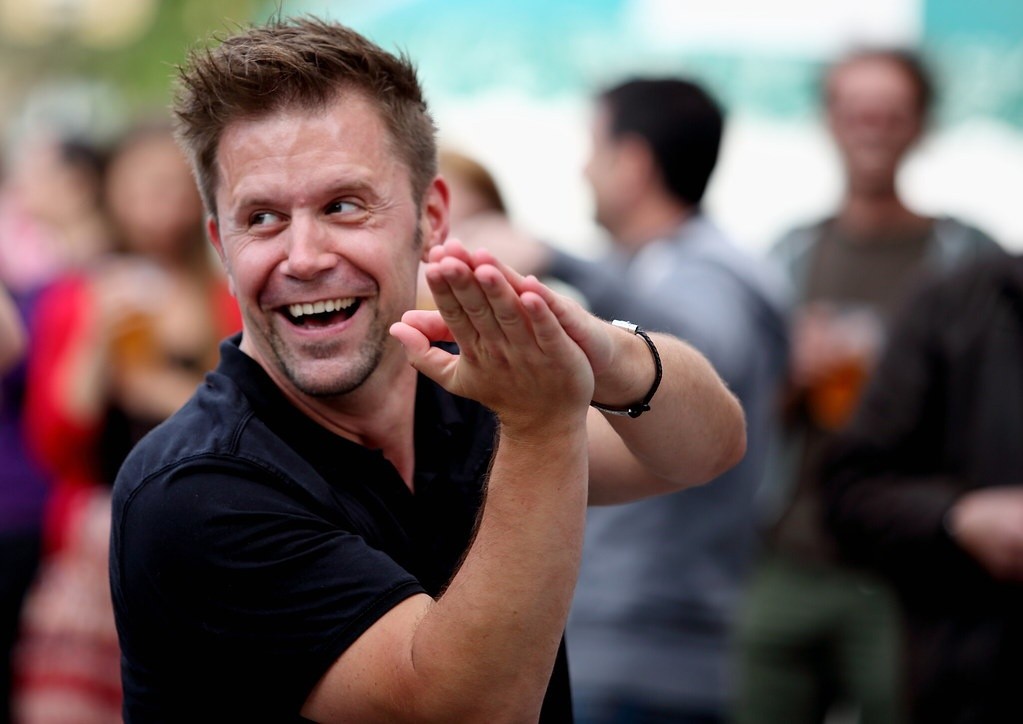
572,319,662,418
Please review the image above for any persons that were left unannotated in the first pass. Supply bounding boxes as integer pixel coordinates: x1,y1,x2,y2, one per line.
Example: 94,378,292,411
103,16,749,723
476,76,791,724
717,49,1023,724
415,139,512,307
0,125,241,724
